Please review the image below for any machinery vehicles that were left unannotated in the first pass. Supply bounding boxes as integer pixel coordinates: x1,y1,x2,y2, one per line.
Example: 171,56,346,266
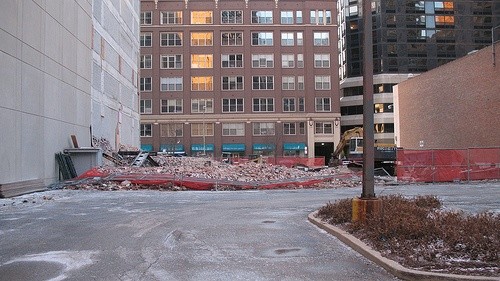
329,127,398,177
